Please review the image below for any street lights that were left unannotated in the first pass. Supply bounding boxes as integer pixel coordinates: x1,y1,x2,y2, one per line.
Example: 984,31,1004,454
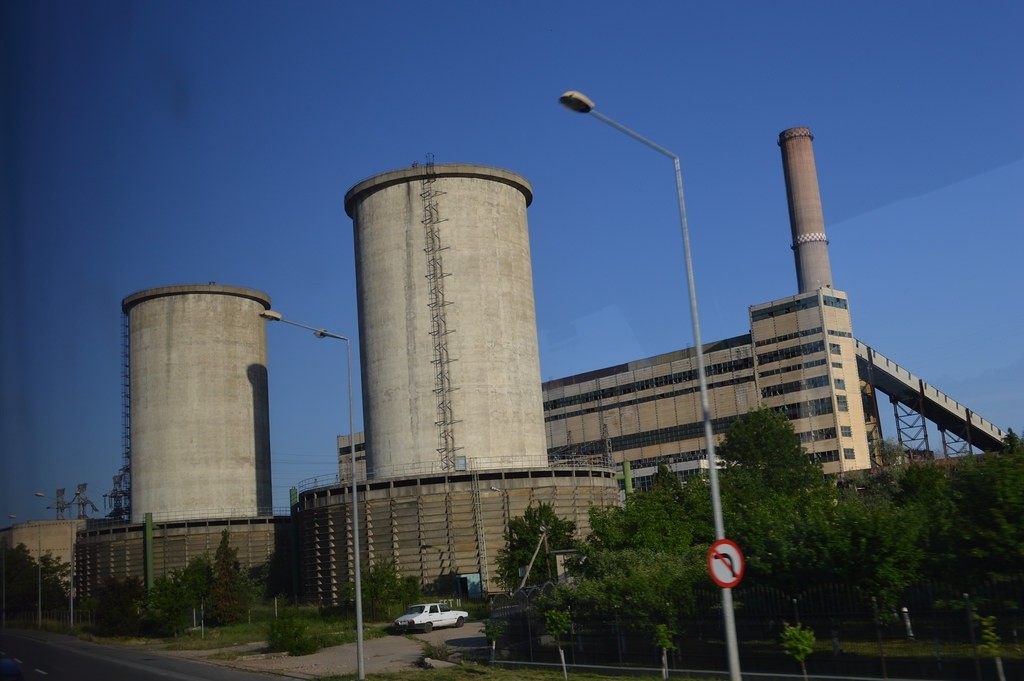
559,87,742,681
8,514,43,629
35,492,74,629
259,309,366,681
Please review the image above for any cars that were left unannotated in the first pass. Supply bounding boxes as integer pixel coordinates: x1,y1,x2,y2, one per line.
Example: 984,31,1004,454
393,602,468,634
